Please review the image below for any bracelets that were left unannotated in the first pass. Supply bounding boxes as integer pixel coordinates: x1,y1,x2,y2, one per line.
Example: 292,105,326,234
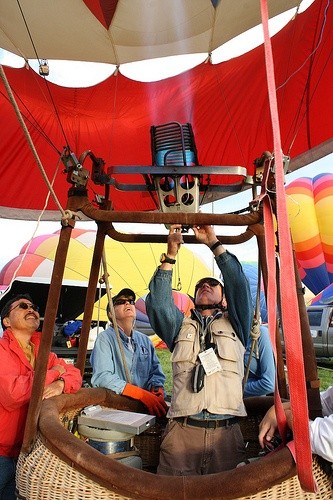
210,241,222,251
57,377,64,381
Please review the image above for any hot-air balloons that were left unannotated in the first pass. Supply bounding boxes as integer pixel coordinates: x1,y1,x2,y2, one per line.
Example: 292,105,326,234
0,0,333,500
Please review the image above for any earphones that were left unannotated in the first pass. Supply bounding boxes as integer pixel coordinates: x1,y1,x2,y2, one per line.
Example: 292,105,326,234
109,312,111,316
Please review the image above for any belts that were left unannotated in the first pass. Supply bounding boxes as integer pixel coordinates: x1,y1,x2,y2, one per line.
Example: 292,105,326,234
173,417,239,428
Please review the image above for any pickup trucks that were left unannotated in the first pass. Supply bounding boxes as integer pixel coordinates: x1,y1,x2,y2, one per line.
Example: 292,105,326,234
261,305,333,367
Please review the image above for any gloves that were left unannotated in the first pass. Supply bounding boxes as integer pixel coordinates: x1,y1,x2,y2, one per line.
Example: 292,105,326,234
120,383,167,418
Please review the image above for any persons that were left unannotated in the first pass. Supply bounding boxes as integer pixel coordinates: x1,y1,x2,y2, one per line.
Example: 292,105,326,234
144,222,255,476
257,385,333,464
243,324,276,397
89,289,168,416
0,294,82,500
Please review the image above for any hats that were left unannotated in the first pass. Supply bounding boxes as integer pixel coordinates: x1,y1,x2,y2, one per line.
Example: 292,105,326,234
106,287,136,323
195,276,224,288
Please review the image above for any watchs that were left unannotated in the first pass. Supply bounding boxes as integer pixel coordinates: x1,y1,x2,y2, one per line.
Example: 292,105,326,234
159,253,176,264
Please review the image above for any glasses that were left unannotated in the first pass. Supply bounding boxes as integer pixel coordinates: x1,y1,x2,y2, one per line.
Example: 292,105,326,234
113,299,134,306
2,302,39,318
196,279,220,288
